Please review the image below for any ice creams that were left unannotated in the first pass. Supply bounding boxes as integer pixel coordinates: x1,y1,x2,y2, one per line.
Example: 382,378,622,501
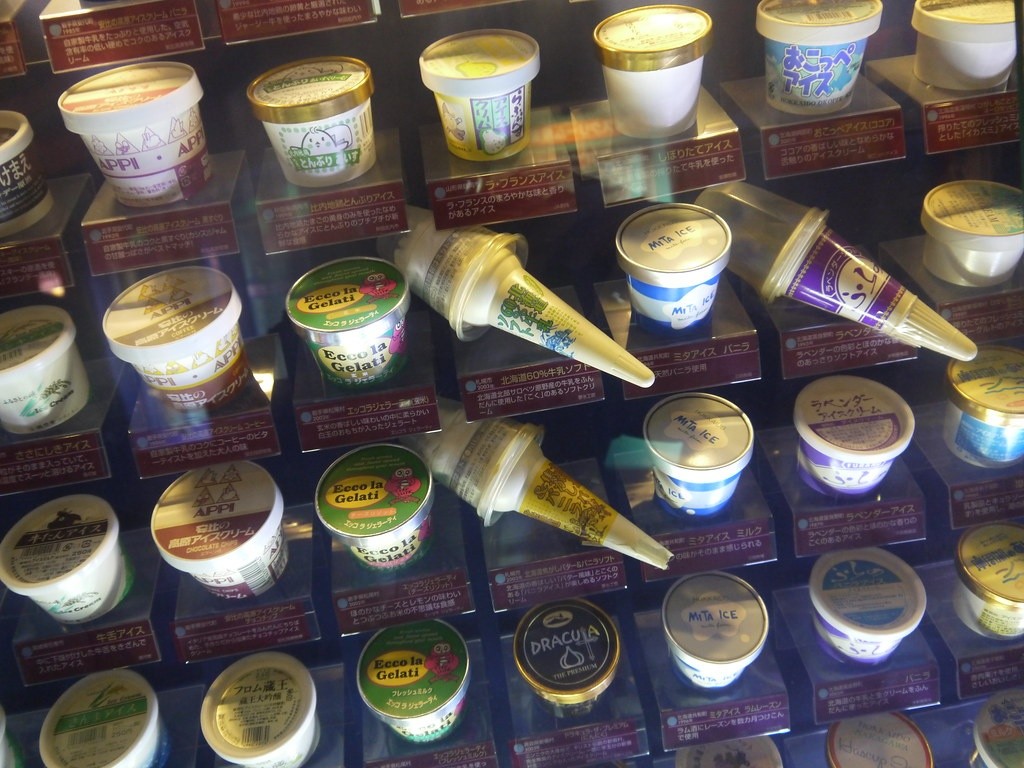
393,211,655,386
694,180,978,361
395,398,675,569
